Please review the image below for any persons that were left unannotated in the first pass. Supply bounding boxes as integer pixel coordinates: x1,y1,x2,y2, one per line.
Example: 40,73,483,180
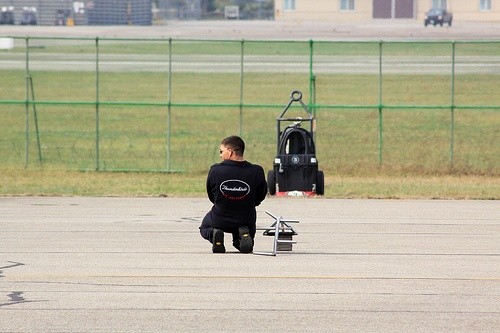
199,134,268,253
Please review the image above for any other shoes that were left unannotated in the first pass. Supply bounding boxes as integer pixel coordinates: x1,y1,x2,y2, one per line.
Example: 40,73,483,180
212,229,225,253
238,226,253,253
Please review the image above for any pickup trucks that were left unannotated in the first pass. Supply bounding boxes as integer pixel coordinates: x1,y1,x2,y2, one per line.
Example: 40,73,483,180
424,7,452,27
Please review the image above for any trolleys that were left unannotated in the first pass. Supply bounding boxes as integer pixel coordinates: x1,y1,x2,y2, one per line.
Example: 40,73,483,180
267,90,325,196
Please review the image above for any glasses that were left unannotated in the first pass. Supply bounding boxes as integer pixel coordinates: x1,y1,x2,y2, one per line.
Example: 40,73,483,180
220,149,230,153
